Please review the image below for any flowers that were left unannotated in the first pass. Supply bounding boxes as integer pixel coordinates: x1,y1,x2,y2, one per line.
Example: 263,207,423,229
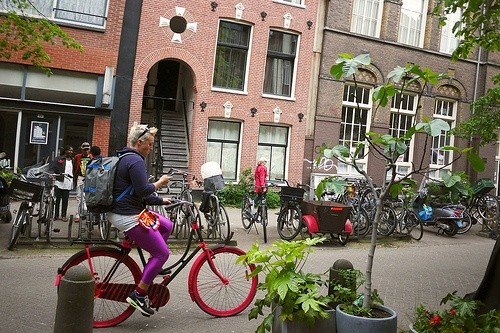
416,294,500,333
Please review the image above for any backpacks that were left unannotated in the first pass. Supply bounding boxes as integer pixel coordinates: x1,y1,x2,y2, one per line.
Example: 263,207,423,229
84,152,144,213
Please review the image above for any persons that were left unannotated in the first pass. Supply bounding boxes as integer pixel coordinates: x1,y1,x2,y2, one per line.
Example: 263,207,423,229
86,146,105,171
49,145,76,222
106,124,173,316
73,142,93,222
247,156,268,215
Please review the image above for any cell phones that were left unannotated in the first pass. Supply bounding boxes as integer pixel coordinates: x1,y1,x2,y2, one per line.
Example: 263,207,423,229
166,168,174,176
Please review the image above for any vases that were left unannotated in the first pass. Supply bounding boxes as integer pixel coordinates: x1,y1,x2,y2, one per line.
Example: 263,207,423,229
409,323,418,333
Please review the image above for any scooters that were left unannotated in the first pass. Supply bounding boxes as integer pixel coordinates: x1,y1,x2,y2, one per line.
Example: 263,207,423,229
404,190,463,236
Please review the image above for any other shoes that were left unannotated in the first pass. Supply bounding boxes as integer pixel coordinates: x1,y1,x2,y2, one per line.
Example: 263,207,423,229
82,215,86,220
54,216,59,221
62,217,68,222
94,220,99,225
75,218,80,222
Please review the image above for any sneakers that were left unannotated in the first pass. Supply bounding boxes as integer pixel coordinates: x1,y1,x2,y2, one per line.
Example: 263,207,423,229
156,268,171,277
126,291,154,316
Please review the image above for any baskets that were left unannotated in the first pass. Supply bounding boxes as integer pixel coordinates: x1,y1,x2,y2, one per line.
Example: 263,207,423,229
281,186,306,202
9,178,44,202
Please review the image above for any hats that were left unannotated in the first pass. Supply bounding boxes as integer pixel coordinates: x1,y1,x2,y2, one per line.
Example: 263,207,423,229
81,142,90,150
258,159,267,165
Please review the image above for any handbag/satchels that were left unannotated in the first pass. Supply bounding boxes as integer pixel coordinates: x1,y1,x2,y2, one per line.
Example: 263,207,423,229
261,184,268,191
419,205,432,220
204,175,227,193
199,193,209,213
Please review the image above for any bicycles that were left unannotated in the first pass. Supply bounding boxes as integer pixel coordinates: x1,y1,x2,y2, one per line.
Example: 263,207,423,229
93,208,111,241
274,178,314,241
441,193,500,232
241,183,274,244
390,198,423,240
147,175,186,238
196,181,230,240
77,178,93,241
166,167,202,241
55,198,258,328
7,171,44,250
334,176,398,237
35,171,73,244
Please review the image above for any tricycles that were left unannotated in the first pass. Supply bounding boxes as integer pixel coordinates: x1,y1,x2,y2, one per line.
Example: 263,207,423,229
300,184,353,246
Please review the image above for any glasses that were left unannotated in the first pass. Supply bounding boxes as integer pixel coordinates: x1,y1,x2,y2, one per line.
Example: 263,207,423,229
137,126,151,140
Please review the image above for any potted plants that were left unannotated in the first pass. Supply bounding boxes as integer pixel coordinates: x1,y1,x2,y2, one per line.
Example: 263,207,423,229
315,53,484,333
240,235,336,333
